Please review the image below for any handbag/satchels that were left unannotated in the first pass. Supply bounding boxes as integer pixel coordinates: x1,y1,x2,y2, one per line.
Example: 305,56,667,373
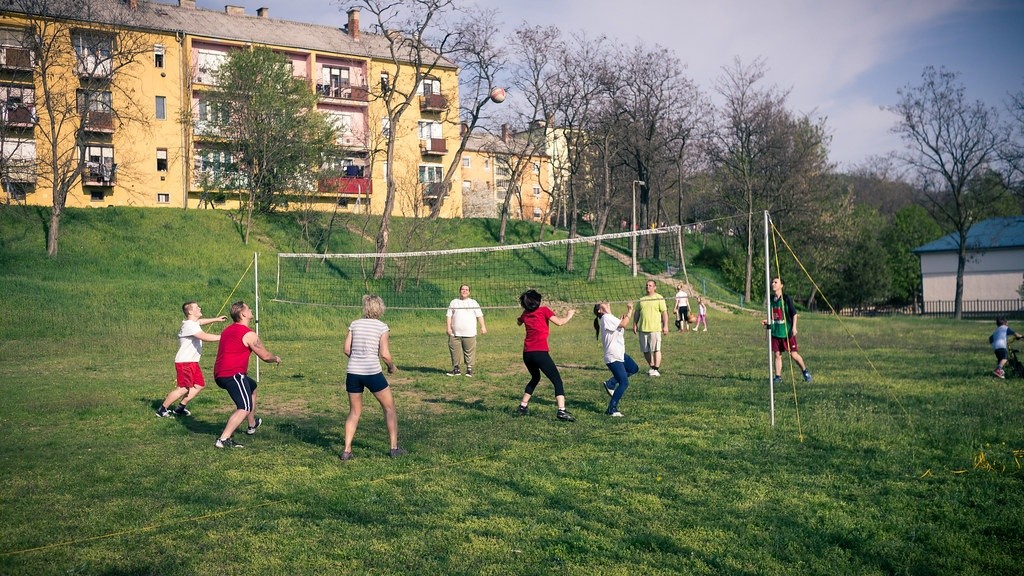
688,310,697,323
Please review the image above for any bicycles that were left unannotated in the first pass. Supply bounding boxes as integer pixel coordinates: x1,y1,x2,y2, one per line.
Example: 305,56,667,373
1006,337,1024,379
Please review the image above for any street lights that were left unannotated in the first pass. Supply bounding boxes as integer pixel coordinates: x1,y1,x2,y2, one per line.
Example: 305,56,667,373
633,180,645,277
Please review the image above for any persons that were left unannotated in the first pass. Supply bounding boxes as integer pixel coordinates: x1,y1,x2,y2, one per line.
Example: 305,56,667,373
340,294,409,460
673,285,691,334
593,301,639,416
155,301,228,418
988,316,1023,379
633,280,669,376
513,289,577,422
692,296,707,331
446,283,488,377
214,301,281,448
673,309,680,330
761,277,811,383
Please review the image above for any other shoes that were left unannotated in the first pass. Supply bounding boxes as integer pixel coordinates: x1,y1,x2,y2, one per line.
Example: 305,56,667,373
556,409,575,422
446,368,461,376
248,418,262,435
517,405,530,415
173,408,190,417
156,408,170,418
774,375,782,382
692,327,698,331
390,446,407,458
609,411,625,417
687,329,689,332
214,436,244,448
802,368,811,382
646,367,660,377
702,328,707,331
464,368,473,377
679,329,683,332
993,367,1006,379
340,449,352,460
604,380,614,397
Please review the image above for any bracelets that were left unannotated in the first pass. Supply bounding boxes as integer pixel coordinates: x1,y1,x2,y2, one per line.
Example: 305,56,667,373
626,314,630,319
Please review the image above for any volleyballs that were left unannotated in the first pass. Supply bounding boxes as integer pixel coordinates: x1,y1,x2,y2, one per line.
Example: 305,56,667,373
490,87,507,103
